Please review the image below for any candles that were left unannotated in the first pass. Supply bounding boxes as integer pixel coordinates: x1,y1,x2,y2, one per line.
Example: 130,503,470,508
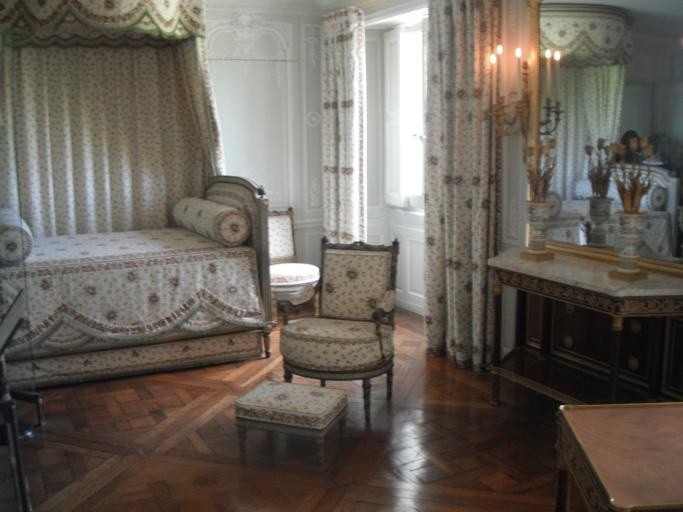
483,44,523,108
543,46,562,102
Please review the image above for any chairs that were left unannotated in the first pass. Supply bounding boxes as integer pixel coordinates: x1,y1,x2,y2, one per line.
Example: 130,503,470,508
547,211,588,247
614,213,675,257
266,210,399,430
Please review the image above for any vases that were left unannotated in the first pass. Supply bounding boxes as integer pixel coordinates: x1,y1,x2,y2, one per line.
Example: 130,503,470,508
525,201,554,250
618,214,649,275
586,194,615,229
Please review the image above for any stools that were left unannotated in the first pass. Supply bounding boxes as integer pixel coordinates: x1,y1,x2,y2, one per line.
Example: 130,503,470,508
232,378,349,463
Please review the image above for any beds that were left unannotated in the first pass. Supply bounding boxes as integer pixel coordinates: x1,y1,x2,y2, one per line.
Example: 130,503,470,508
0,175,274,390
556,164,677,257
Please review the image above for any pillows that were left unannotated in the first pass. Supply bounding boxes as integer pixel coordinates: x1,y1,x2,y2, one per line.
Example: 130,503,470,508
572,179,668,209
170,197,249,247
0,204,33,267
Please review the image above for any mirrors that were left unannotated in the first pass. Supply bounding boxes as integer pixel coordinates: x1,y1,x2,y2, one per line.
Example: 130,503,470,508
527,2,682,281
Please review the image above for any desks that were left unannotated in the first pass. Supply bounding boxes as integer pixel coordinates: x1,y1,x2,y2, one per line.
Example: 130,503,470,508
484,247,683,418
552,402,683,512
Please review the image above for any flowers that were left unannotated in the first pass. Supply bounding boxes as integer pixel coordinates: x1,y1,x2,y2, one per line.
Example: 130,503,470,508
523,137,559,200
609,138,655,211
584,137,617,197
